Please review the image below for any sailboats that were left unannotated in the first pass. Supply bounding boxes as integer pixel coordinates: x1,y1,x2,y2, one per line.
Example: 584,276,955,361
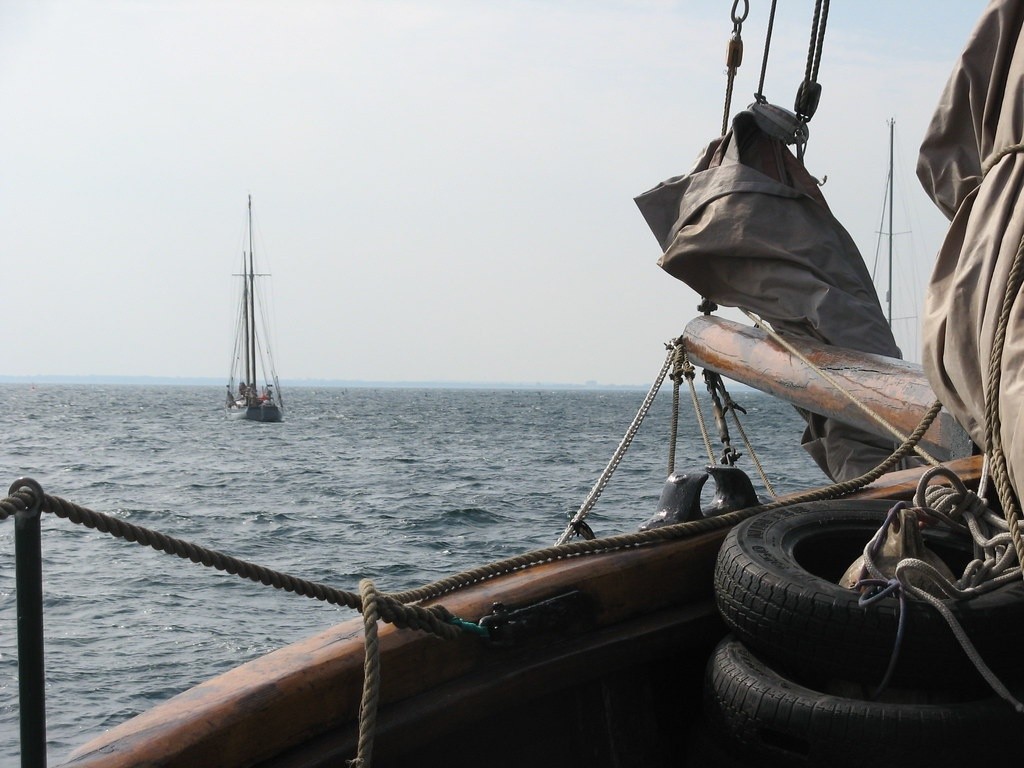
223,194,284,421
